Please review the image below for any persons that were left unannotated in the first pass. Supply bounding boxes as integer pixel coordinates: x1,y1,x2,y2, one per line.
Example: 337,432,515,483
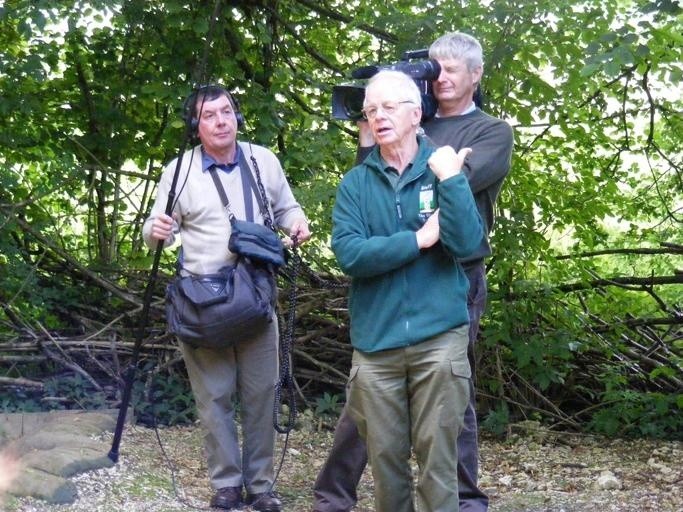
139,83,317,512
305,30,513,510
326,66,489,511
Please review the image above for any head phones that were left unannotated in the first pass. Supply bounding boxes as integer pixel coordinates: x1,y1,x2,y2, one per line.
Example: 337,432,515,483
183,88,249,130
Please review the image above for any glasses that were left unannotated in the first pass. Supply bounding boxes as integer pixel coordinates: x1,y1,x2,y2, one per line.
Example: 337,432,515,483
361,100,417,120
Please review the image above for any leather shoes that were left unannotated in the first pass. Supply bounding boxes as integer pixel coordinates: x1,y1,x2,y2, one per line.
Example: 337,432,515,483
245,490,283,512
210,484,243,508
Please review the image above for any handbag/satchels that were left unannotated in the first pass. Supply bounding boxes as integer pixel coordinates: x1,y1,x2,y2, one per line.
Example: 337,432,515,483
200,144,285,270
165,245,278,349
